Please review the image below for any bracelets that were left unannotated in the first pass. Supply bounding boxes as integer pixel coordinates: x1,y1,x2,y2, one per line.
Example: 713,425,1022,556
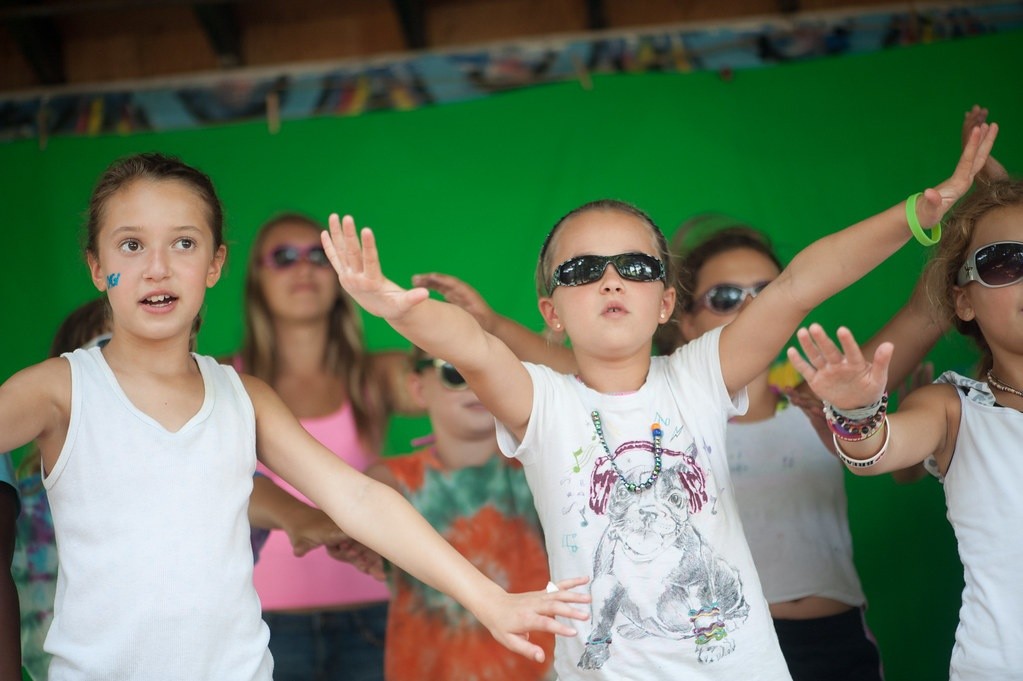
819,390,892,469
906,191,941,246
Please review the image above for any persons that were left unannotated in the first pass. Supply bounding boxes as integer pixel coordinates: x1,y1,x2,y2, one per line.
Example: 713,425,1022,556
785,103,1023,681
317,121,1000,681
0,150,594,681
409,224,953,681
893,360,940,484
0,211,557,680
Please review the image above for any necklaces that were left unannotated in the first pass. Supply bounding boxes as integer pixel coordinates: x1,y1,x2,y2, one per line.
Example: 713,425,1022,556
986,366,1023,398
728,384,790,432
591,410,664,493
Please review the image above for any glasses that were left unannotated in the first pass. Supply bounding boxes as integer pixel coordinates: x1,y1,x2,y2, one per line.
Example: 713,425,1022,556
414,360,469,391
260,245,331,268
957,241,1023,288
548,250,670,297
688,280,772,315
